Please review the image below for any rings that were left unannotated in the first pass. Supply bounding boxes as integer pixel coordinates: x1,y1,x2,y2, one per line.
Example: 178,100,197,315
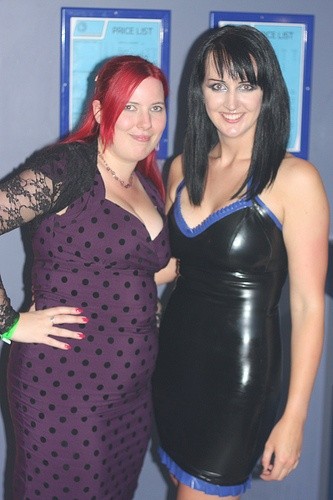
50,315,56,324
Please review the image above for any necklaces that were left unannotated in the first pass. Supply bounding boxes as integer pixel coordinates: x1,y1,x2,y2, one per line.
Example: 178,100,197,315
98,152,134,188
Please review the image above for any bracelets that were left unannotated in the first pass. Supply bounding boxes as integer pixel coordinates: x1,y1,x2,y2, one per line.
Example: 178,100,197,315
0,311,20,345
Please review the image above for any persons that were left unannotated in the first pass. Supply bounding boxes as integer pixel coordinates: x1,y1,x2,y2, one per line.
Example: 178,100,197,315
146,22,330,500
0,53,176,500
